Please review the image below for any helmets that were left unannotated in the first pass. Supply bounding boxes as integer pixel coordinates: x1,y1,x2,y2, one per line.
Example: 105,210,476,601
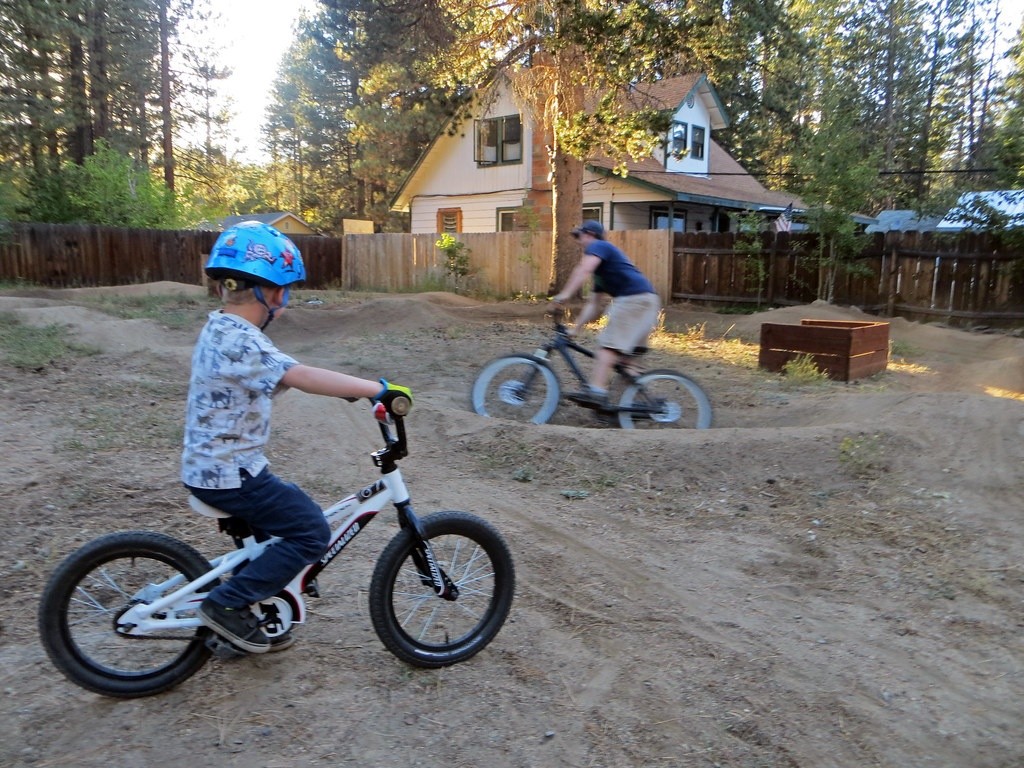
204,219,307,290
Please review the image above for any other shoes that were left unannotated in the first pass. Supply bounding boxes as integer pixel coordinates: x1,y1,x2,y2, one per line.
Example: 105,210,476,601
560,384,608,407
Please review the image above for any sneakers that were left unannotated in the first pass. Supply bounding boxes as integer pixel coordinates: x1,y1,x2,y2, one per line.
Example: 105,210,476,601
194,595,272,656
266,632,296,653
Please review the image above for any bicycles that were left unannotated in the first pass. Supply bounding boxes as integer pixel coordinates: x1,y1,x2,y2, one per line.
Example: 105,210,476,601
36,392,515,700
472,301,712,431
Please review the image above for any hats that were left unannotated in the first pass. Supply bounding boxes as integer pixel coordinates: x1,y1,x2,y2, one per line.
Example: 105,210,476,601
568,219,605,240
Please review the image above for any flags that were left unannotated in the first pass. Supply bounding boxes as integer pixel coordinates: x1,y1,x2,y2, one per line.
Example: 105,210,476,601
775,203,792,233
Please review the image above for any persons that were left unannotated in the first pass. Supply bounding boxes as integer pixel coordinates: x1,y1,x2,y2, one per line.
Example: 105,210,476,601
544,221,661,409
181,221,415,654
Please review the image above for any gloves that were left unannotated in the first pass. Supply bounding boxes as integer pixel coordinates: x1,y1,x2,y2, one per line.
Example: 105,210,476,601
373,377,414,421
